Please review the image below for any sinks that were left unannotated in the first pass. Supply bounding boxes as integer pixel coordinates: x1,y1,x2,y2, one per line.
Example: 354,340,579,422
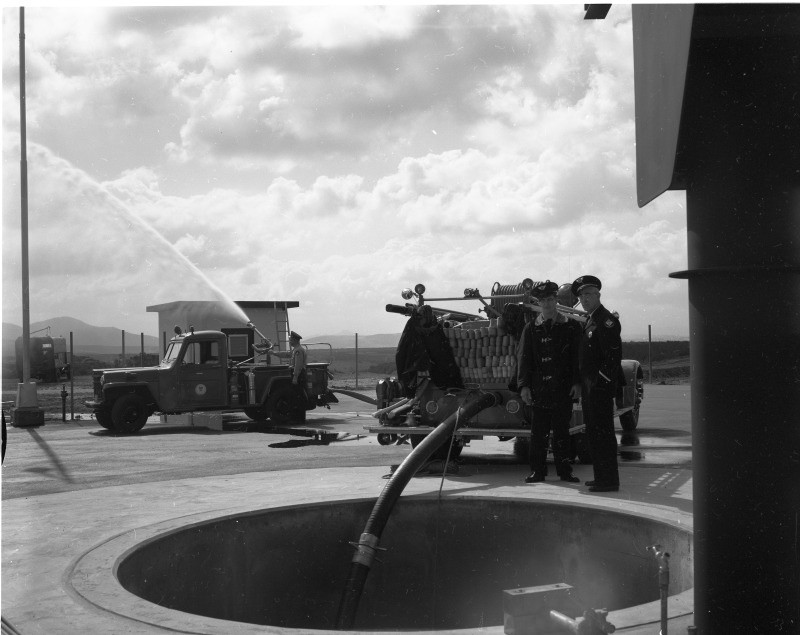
106,491,694,633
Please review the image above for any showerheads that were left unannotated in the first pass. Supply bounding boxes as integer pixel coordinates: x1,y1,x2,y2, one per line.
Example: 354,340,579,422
246,320,256,330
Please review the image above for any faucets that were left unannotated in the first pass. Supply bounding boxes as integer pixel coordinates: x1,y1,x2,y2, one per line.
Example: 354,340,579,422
646,543,671,572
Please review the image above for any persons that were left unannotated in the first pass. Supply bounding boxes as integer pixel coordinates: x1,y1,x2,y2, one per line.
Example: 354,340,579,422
515,283,583,484
268,331,308,423
570,276,626,492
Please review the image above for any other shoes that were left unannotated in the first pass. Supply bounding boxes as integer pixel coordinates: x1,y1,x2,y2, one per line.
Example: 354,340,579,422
589,486,619,492
586,480,596,486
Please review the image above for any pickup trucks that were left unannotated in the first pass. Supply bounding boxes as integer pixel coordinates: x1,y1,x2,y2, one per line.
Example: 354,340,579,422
361,354,646,463
83,325,339,434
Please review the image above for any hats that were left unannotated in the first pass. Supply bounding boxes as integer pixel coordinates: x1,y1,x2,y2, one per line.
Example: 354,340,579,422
571,276,601,297
289,331,302,340
531,282,559,302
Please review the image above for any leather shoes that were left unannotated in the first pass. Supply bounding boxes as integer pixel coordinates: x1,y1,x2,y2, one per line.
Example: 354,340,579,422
525,472,545,483
560,475,580,483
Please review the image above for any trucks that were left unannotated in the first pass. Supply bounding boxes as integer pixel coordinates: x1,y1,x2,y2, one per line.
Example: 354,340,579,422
15,336,68,383
